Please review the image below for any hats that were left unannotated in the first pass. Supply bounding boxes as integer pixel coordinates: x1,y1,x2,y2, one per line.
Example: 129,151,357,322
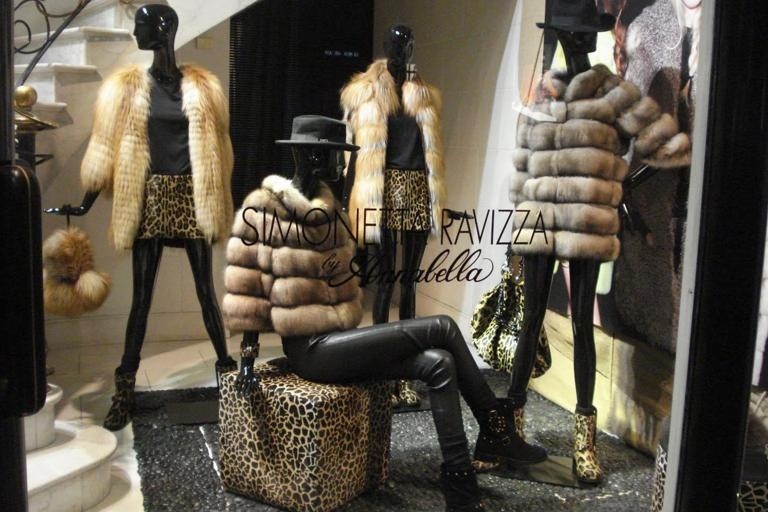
275,114,361,154
535,0,616,34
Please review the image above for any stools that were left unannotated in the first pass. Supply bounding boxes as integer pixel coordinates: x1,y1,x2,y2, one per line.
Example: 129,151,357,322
217,355,394,512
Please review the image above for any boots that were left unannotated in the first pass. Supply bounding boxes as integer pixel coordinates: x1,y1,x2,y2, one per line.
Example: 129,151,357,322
390,380,423,408
441,461,487,512
104,366,138,431
474,399,548,471
571,405,606,486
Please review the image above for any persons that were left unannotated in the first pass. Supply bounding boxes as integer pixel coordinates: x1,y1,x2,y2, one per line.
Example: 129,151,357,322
497,1,694,490
338,23,448,410
220,112,552,512
42,4,237,429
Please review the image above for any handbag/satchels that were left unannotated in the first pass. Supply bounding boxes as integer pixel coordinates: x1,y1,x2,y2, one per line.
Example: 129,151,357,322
42,228,111,319
471,277,552,380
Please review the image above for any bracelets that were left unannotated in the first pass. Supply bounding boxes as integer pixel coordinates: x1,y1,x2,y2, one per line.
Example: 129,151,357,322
239,340,260,359
